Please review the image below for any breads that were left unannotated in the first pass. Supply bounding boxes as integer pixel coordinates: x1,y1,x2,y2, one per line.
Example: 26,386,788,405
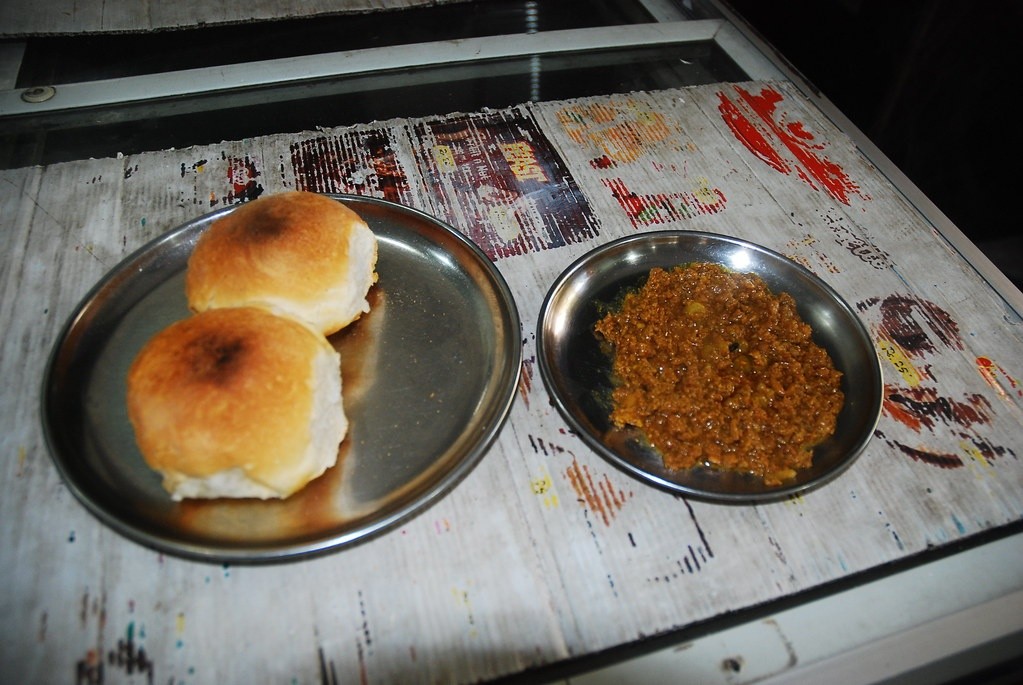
185,191,379,337
125,306,350,502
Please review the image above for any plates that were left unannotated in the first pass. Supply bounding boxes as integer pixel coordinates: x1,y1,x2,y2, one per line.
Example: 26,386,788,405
533,227,887,505
36,193,524,568
